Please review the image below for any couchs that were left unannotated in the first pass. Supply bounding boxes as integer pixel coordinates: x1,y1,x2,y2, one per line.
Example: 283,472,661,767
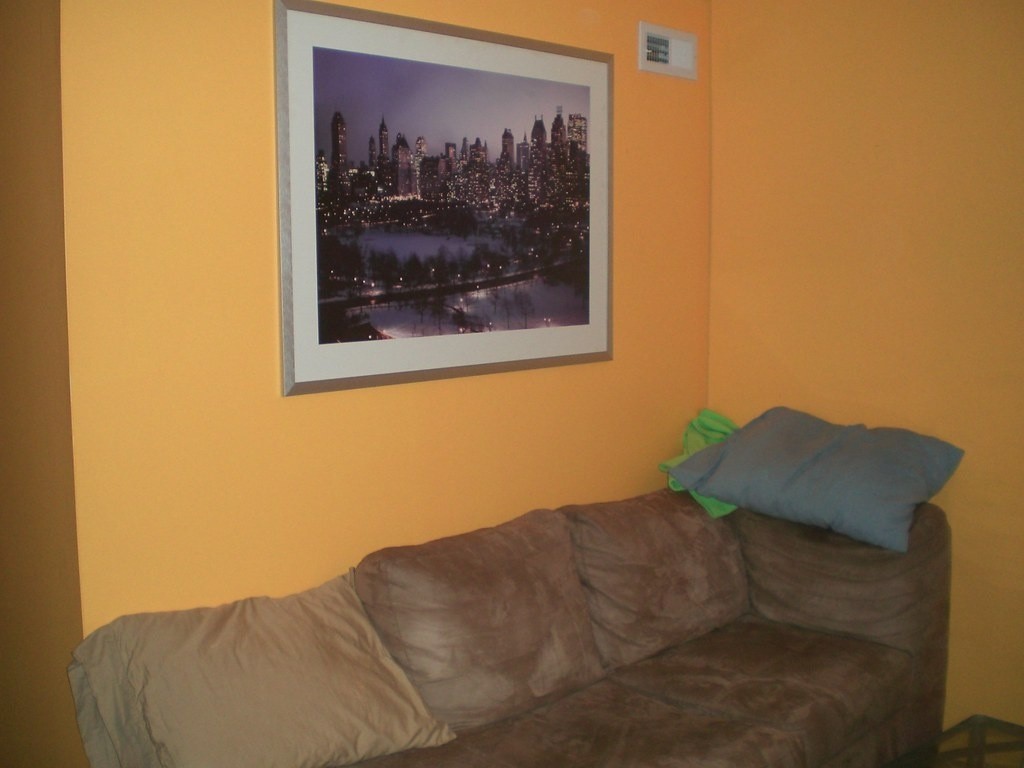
68,407,953,768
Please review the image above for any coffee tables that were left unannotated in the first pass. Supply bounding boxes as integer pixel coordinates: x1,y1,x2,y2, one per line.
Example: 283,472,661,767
886,714,1024,768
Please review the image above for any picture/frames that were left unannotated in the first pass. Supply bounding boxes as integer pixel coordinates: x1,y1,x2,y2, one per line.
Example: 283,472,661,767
273,0,613,397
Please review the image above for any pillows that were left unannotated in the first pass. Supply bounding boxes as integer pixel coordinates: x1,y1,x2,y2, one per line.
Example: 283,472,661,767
358,510,609,740
558,490,750,668
674,408,962,552
77,575,458,768
